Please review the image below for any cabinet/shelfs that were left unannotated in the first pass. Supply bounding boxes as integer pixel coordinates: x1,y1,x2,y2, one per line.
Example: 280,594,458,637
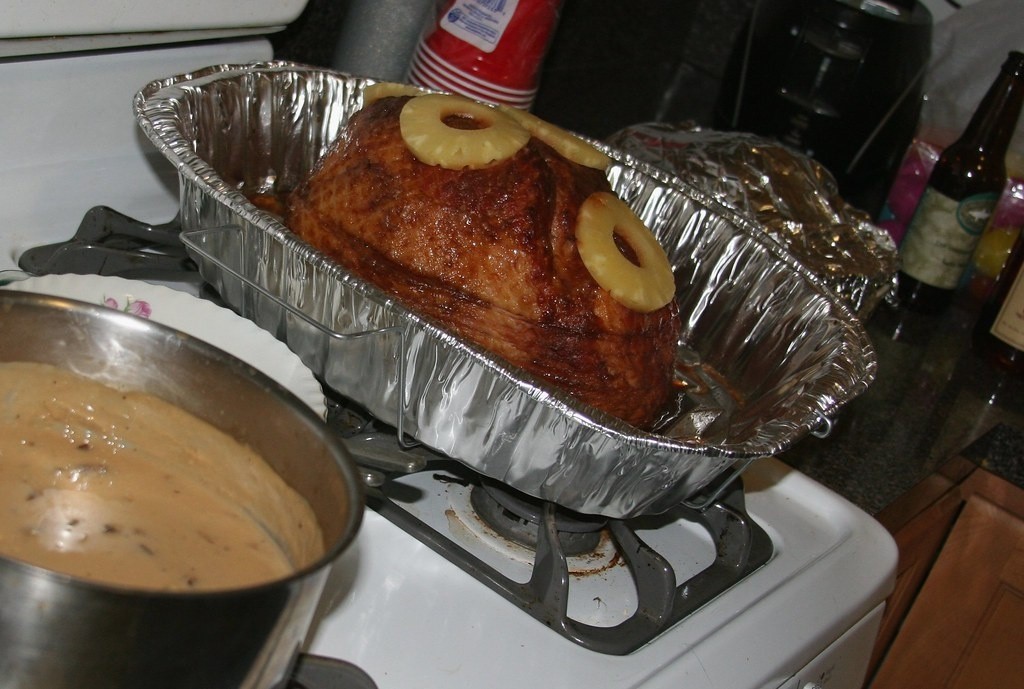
865,486,1024,689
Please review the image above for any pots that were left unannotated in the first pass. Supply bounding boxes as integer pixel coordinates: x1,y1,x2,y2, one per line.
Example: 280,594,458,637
132,59,878,518
0,288,366,689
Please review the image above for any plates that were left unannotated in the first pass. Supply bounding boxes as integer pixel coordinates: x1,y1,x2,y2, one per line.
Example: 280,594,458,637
0,273,328,425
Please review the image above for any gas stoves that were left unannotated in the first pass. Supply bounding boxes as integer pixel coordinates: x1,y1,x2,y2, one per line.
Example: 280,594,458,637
0,205,898,689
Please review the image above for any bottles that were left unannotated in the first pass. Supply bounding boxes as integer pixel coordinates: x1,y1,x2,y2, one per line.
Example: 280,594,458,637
879,51,1024,309
978,223,1024,375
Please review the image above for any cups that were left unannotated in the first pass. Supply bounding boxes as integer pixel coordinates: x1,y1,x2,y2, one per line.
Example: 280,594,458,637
402,1,557,114
